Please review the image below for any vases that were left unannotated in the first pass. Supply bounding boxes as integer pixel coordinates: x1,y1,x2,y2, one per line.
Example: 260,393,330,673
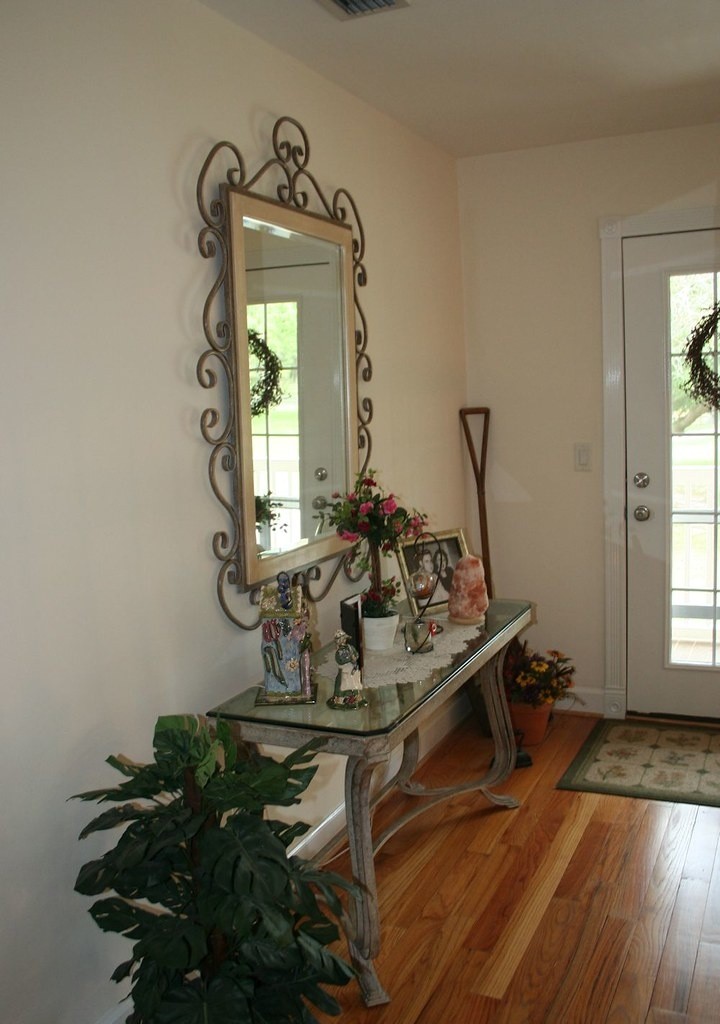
507,704,553,744
362,609,400,651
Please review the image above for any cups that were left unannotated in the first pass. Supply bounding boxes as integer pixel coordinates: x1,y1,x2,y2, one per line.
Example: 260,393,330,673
257,608,311,703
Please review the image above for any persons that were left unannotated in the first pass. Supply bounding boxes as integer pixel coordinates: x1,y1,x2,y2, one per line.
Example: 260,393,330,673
413,548,454,607
326,629,369,711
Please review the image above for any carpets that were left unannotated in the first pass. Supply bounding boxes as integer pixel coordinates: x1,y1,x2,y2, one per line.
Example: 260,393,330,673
554,719,720,809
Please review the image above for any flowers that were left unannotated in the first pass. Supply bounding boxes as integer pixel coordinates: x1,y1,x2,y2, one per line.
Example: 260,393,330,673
313,468,430,614
502,650,575,709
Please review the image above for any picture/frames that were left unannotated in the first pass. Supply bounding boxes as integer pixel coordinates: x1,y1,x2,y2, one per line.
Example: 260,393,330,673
394,526,468,617
340,594,363,669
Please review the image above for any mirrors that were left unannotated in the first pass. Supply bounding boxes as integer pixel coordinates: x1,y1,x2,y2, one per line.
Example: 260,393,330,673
196,113,375,631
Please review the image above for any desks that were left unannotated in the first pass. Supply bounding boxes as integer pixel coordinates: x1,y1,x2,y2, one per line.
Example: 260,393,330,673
204,593,538,1007
